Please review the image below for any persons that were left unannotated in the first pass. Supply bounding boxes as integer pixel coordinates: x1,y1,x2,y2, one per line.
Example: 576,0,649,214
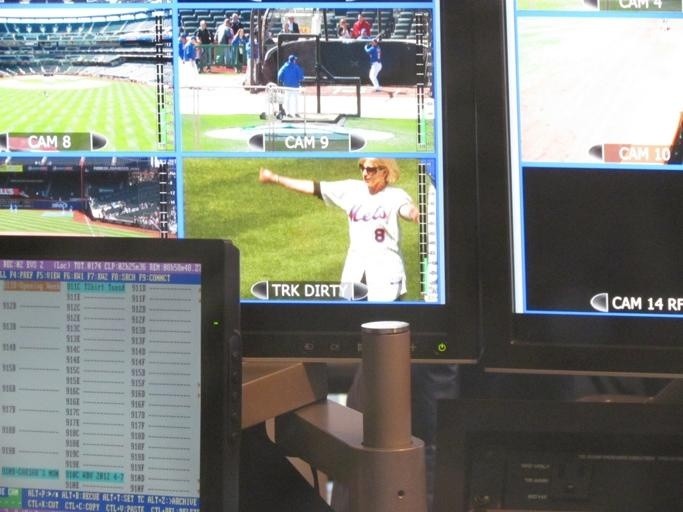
91,195,160,229
258,157,419,303
180,11,384,117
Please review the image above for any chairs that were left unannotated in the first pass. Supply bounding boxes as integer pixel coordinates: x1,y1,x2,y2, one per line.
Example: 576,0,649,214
178,8,432,99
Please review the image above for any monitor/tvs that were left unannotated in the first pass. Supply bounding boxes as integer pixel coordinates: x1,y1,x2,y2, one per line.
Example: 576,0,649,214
0,234,242,512
479,0,683,381
0,0,484,368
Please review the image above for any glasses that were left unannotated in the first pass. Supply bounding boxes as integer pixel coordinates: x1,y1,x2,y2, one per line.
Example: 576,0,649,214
359,163,386,175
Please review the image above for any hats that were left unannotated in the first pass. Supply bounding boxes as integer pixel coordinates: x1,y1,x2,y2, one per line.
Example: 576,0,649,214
289,55,298,61
181,33,186,39
191,36,197,40
